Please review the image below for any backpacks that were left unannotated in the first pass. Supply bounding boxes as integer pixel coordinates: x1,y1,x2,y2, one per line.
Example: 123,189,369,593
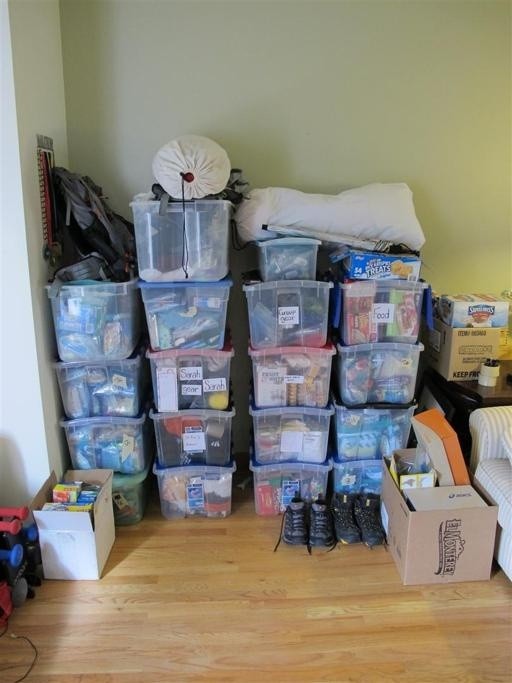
50,166,137,279
152,169,249,202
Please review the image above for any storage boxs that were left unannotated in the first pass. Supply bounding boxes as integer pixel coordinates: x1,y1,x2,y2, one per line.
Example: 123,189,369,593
423,314,510,381
335,238,420,283
249,446,332,516
152,463,238,520
328,456,384,508
242,280,333,349
31,469,115,581
329,270,433,344
51,356,143,419
380,448,499,586
329,338,424,405
437,291,511,327
67,464,147,525
255,237,322,282
247,392,335,465
145,348,236,410
133,282,234,352
43,277,143,363
410,407,469,486
149,409,236,468
246,341,337,408
128,194,233,283
60,414,156,474
331,402,417,461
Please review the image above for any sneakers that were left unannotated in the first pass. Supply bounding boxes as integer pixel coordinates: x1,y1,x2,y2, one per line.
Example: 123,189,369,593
281,491,386,548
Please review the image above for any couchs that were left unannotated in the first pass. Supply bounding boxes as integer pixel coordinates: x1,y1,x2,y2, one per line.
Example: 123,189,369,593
468,405,511,588
